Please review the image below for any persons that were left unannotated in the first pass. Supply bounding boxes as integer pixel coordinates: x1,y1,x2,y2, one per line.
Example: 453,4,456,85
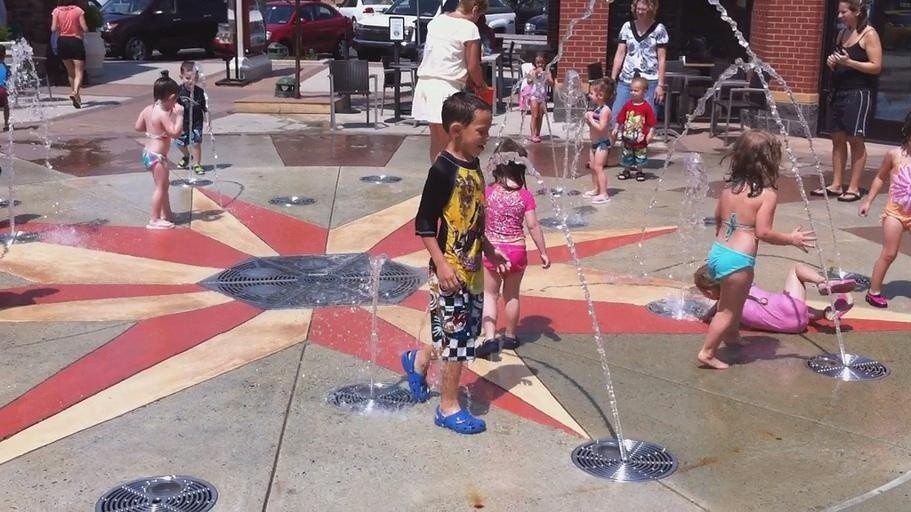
856,111,911,308
695,263,857,334
0,44,15,131
696,129,818,369
173,61,208,175
810,1,883,203
49,0,89,108
134,70,184,230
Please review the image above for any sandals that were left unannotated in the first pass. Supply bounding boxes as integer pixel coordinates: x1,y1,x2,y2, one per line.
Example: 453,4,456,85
633,172,646,182
527,134,542,144
473,334,521,358
864,290,888,309
616,169,632,181
399,348,433,405
816,277,857,322
431,402,487,437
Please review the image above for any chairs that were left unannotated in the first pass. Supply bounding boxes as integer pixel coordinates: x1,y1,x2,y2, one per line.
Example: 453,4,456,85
584,55,772,147
379,27,518,119
324,56,381,132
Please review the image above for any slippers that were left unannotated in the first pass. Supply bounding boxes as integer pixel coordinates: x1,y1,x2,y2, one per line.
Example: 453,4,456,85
580,190,611,204
159,212,180,220
143,219,174,231
807,183,863,203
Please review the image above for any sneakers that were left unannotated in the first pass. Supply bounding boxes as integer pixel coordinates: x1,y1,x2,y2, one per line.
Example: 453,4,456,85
176,154,193,169
68,91,81,109
190,163,207,175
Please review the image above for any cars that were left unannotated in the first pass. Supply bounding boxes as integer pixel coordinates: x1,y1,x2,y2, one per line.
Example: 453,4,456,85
879,9,910,50
